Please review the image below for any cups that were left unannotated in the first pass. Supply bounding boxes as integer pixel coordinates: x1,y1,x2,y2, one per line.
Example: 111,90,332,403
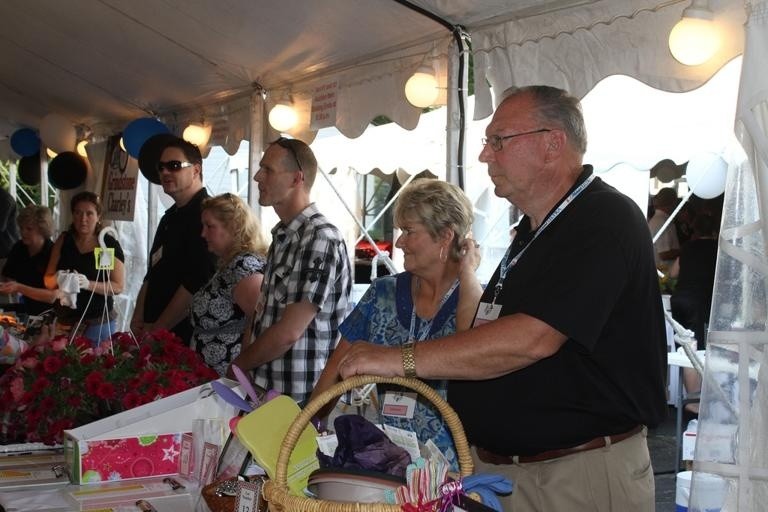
8,291,20,303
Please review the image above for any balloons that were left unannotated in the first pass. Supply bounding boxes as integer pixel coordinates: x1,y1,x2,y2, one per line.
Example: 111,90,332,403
37,111,76,154
43,149,87,189
9,128,40,155
122,114,173,158
16,157,40,187
0,138,24,163
137,133,180,186
678,152,731,203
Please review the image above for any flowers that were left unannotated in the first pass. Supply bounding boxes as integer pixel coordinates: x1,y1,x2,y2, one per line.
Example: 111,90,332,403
1,326,220,449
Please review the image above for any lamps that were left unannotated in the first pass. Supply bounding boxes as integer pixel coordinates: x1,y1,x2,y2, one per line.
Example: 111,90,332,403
403,48,440,109
666,1,724,67
183,108,213,150
267,81,301,132
76,122,97,157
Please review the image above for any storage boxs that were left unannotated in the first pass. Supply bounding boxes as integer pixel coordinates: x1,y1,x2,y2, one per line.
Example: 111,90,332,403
678,414,738,468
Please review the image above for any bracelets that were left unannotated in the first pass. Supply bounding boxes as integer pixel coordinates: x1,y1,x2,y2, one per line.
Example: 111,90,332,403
400,342,418,381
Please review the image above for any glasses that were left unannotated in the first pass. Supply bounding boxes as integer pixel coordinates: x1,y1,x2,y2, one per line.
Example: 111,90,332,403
274,132,305,184
480,128,553,152
156,157,202,174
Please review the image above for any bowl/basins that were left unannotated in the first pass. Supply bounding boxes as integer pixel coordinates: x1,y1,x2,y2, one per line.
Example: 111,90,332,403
306,468,408,505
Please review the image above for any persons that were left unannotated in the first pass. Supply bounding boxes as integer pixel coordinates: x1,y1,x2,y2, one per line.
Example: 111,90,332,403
0,175,24,264
334,83,666,512
130,138,215,349
42,190,124,349
647,187,725,290
225,136,355,417
670,215,717,414
185,190,268,382
0,205,56,317
306,178,484,477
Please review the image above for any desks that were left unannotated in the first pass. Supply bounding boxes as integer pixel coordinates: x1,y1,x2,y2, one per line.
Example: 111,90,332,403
665,348,766,500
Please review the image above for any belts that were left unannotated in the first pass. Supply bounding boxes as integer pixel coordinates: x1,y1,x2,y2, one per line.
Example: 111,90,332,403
461,414,646,465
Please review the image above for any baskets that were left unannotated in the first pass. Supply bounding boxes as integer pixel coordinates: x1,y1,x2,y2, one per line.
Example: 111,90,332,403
260,369,480,512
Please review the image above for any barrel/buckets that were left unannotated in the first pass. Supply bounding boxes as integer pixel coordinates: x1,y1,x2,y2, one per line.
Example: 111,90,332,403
676,469,725,512
